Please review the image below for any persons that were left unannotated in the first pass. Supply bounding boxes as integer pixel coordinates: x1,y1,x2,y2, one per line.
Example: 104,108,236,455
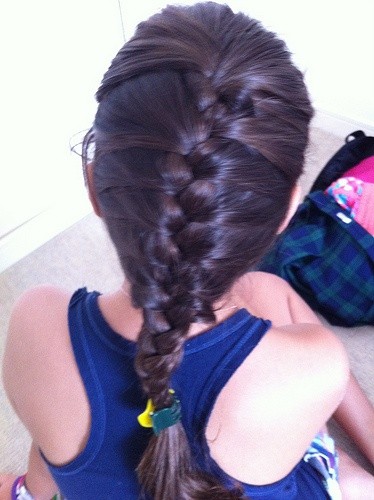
0,0,374,500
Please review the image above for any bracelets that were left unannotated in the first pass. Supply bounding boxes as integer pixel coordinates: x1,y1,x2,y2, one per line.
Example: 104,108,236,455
11,476,33,500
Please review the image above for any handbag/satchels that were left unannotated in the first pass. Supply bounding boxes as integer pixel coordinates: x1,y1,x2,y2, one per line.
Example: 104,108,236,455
258,129,374,328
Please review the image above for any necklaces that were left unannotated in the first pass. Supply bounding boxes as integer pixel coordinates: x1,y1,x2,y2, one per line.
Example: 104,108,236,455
119,288,239,313
